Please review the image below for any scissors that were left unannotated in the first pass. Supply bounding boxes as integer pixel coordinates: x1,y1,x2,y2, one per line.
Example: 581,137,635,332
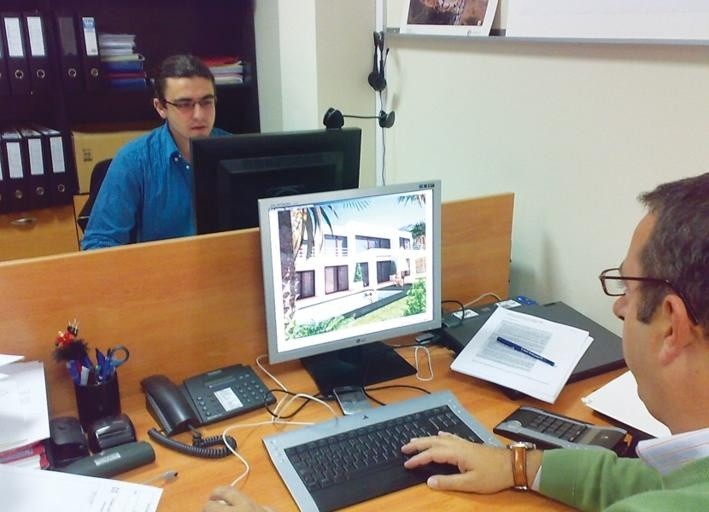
107,346,129,373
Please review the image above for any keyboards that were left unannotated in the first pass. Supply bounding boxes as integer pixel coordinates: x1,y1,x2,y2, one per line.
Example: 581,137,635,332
261,389,505,512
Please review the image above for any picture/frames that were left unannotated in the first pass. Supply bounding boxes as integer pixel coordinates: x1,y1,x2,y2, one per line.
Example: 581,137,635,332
399,0,498,36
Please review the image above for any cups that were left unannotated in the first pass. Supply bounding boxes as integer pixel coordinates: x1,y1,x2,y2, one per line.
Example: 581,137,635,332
74,372,123,437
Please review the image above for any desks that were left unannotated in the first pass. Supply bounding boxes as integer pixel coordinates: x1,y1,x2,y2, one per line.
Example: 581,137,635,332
0,333,628,512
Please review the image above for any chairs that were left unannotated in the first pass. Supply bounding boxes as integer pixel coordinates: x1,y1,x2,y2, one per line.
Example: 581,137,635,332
79,158,115,236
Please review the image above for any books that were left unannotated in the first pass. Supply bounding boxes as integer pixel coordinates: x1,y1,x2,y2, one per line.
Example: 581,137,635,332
96,33,147,90
450,306,595,405
201,57,244,86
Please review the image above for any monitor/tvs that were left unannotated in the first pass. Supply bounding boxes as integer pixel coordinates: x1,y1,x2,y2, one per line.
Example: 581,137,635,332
257,179,442,398
189,126,362,235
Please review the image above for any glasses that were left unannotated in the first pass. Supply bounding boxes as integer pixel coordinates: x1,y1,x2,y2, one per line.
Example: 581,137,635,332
599,268,700,327
165,95,217,110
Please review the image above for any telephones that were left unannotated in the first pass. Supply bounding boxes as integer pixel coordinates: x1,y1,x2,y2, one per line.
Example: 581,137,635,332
142,361,274,457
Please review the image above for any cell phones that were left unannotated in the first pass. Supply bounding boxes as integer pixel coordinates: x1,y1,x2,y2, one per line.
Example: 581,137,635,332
332,386,373,416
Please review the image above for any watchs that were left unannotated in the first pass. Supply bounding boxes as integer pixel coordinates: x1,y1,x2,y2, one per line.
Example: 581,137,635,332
507,441,536,492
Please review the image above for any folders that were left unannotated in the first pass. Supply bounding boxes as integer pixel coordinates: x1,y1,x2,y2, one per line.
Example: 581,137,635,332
0,9,100,96
0,125,69,213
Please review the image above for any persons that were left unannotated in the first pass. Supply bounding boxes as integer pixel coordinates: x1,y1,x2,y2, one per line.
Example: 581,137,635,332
79,54,233,251
400,172,709,512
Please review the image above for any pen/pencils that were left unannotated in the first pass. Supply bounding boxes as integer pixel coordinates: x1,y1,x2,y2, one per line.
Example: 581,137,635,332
498,335,553,366
67,347,113,383
